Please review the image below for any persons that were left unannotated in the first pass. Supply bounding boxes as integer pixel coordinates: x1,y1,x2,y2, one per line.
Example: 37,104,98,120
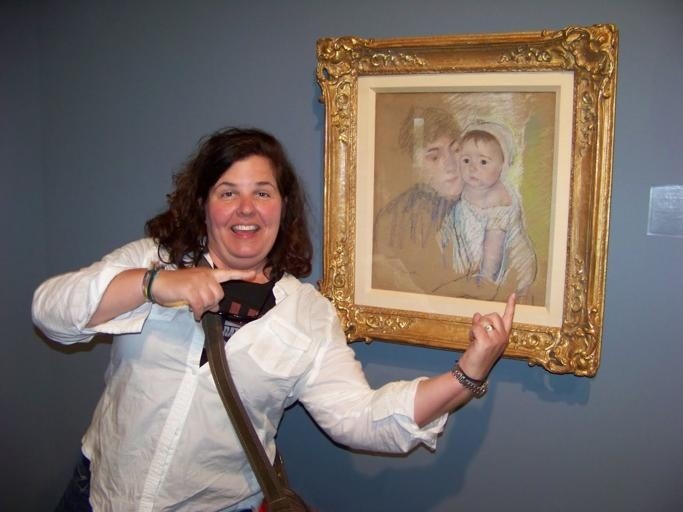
30,125,516,511
370,106,494,301
433,122,537,300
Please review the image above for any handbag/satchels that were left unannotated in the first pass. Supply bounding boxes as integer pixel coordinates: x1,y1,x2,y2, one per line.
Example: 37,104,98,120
266,477,314,511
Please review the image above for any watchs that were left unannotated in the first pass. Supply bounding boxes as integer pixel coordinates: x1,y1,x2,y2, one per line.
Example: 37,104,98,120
450,362,489,399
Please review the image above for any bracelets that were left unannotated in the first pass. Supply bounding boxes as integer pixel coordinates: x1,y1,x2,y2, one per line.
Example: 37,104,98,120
140,266,164,303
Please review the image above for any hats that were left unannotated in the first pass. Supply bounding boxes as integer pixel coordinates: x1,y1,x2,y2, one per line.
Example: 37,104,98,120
462,116,518,167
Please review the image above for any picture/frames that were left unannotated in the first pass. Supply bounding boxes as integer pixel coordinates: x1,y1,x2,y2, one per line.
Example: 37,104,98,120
313,22,617,379
